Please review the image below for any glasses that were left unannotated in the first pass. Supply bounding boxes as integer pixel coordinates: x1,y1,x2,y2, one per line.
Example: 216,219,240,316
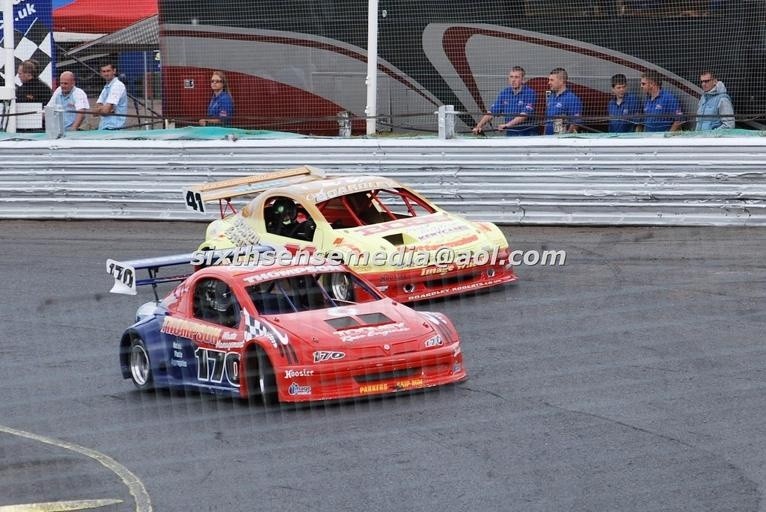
700,79,712,83
211,79,221,82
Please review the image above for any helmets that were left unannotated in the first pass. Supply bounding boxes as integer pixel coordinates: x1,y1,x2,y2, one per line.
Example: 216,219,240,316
272,199,298,225
206,282,232,312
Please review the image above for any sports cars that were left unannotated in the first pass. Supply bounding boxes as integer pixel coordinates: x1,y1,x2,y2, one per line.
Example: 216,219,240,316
106,245,466,406
183,164,517,305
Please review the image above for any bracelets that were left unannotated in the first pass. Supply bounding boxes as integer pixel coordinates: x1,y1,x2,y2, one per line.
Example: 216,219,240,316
205,118,209,125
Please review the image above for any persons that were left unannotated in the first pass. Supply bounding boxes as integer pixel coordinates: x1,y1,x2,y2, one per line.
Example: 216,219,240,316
544,68,583,135
14,58,52,104
200,72,234,128
94,61,128,130
268,198,299,235
203,279,232,327
472,65,538,135
42,71,92,131
695,71,735,130
608,74,642,132
640,72,681,132
